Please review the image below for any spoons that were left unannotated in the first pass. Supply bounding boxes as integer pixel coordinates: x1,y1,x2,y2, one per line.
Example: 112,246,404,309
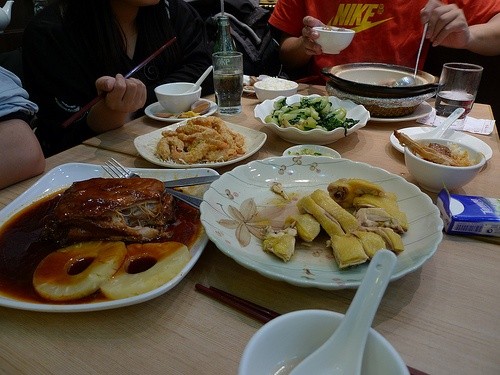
418,108,465,139
290,249,397,375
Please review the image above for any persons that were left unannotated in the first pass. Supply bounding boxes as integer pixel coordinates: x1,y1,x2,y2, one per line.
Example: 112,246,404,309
267,0,500,86
22,0,214,157
0,66,45,190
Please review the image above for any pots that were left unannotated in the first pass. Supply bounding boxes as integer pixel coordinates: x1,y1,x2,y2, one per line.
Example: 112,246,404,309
321,61,438,118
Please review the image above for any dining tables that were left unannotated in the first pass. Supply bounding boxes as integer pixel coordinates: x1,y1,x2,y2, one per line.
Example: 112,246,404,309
0,84,500,375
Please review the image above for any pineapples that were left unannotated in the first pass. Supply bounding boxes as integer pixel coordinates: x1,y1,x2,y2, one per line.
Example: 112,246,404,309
101,241,191,299
33,240,126,301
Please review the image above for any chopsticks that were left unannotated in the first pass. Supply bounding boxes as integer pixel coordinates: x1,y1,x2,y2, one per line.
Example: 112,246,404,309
196,284,428,375
62,35,175,128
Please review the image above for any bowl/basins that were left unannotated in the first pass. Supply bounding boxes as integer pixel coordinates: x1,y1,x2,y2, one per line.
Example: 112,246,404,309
155,81,201,112
239,309,410,375
254,78,299,102
312,25,355,53
406,140,486,195
254,92,371,145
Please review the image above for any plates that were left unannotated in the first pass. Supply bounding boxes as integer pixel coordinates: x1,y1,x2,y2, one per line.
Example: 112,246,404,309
0,162,220,312
135,117,267,169
391,127,493,160
368,102,433,123
243,83,256,93
200,154,444,290
282,145,341,158
145,98,218,122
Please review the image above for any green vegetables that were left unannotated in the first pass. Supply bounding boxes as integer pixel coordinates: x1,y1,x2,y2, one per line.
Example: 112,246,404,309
265,96,360,137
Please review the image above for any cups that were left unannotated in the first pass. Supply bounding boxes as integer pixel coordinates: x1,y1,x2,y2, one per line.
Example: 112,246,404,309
434,62,484,128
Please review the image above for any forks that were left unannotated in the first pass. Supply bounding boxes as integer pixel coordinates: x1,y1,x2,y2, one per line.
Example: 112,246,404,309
101,158,203,209
